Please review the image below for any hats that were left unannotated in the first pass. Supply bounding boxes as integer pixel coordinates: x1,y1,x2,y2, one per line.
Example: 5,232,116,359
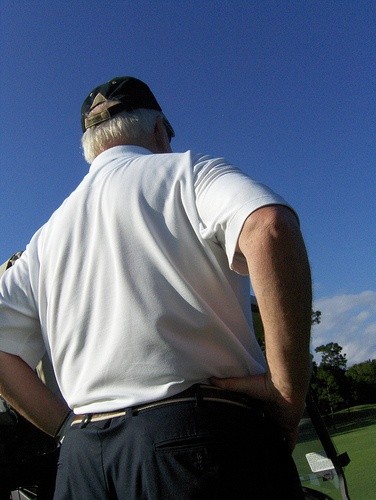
81,76,175,138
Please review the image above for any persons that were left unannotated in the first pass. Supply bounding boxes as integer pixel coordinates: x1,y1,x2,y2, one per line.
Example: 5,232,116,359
0,76,333,500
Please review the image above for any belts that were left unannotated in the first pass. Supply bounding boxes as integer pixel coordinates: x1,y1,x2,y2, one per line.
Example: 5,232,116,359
70,384,268,428
